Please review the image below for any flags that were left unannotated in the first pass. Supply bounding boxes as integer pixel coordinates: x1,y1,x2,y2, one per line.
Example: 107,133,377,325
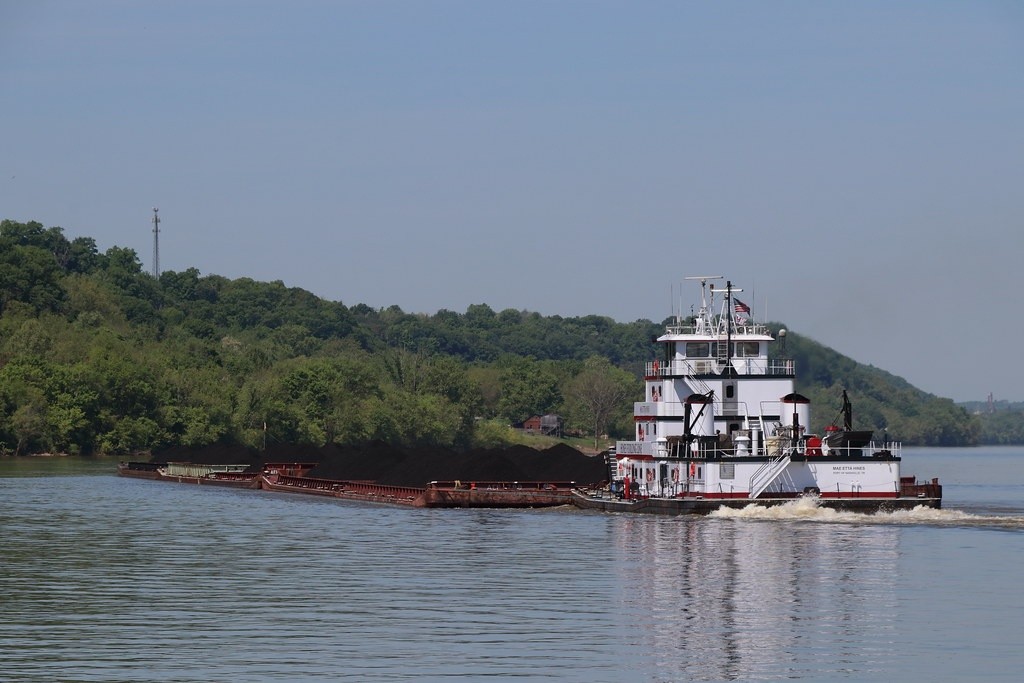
733,297,750,317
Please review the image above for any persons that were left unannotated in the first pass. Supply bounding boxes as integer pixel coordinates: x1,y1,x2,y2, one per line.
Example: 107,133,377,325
687,459,695,480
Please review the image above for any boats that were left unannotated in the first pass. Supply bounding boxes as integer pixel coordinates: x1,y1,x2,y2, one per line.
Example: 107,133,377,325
568,274,945,514
265,463,320,477
261,473,425,507
426,480,576,505
118,462,261,489
